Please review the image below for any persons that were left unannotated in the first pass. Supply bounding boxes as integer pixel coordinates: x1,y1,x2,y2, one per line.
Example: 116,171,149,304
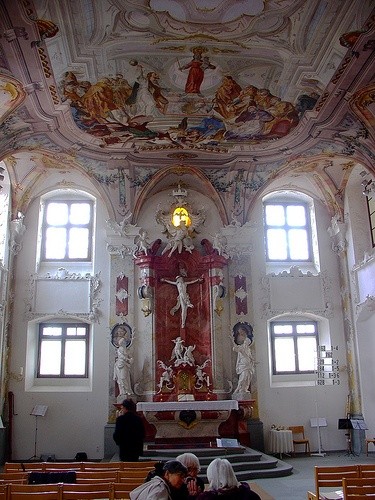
138,231,151,255
168,453,205,500
128,459,188,500
195,458,261,500
113,399,146,462
232,338,255,394
174,337,196,367
160,276,203,328
113,338,134,397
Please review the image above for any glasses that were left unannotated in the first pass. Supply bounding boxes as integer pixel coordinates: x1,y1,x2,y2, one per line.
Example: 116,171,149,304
186,467,199,472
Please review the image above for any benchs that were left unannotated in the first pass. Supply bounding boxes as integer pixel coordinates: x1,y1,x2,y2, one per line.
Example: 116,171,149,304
307,464,375,500
0,460,159,500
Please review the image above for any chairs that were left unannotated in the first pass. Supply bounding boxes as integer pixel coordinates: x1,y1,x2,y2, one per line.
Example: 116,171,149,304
289,426,311,457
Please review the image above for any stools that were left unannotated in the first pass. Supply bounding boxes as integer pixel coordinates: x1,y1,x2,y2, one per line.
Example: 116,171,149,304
366,440,375,457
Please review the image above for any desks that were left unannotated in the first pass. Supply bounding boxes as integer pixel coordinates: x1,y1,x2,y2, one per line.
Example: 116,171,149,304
269,430,293,459
136,400,238,448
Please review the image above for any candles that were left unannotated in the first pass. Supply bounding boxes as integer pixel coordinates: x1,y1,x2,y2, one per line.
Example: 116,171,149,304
207,376,209,386
161,377,162,387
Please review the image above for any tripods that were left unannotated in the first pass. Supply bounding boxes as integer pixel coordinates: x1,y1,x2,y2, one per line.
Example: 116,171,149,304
25,405,48,462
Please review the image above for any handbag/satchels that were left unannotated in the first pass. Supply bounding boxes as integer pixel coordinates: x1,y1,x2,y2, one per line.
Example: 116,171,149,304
74,452,88,462
